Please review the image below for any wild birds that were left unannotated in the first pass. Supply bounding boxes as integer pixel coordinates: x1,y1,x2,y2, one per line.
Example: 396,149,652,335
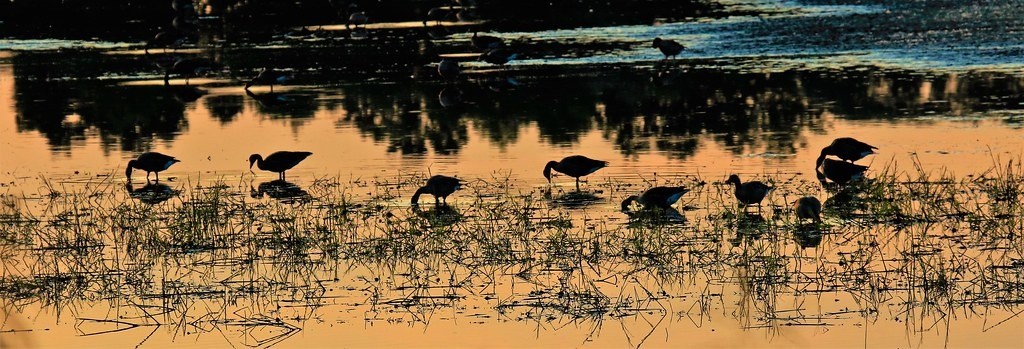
726,172,774,218
411,172,462,211
814,131,877,169
541,152,609,193
620,182,692,216
652,37,687,60
123,147,180,182
791,196,824,226
127,1,535,116
813,154,867,189
247,146,314,185
